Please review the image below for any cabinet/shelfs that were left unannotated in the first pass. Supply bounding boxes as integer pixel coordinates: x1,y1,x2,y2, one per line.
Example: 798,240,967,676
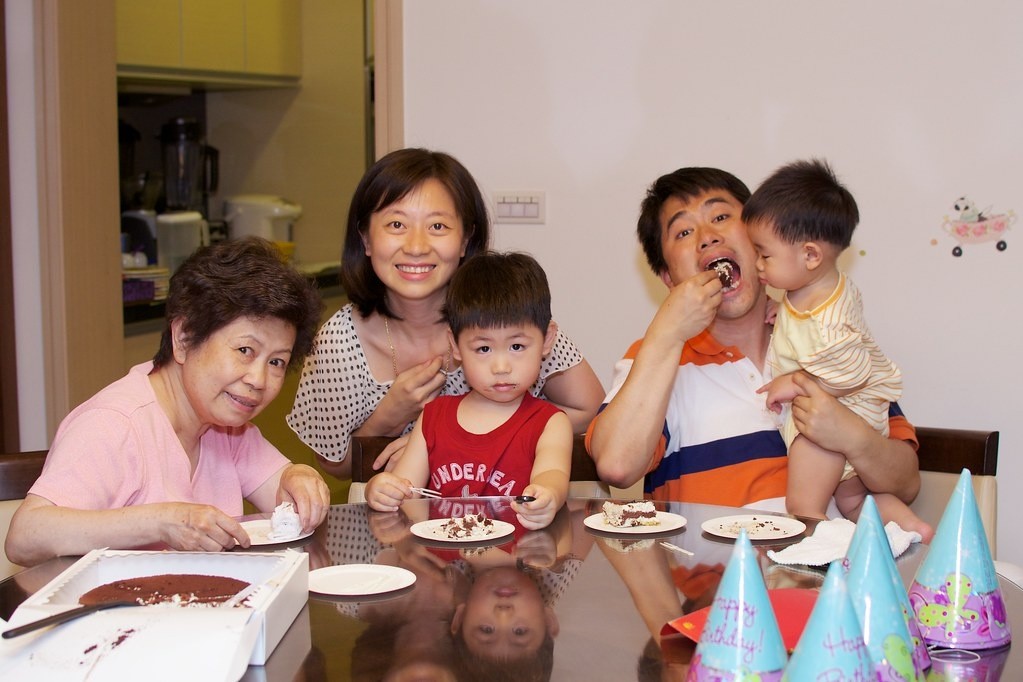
113,0,302,94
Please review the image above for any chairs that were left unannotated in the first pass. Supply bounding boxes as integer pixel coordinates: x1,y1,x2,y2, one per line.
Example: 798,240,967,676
883,428,997,566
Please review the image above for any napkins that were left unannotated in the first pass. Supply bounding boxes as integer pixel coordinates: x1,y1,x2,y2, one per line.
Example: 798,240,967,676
764,517,921,566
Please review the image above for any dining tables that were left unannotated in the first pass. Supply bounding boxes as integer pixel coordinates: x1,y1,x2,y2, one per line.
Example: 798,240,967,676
0,495,1023,682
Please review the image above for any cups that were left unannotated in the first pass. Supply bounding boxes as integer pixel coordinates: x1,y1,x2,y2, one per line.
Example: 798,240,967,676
121,252,148,269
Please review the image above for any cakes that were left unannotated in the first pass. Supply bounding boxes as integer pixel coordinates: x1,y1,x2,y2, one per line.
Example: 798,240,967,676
712,262,733,289
267,503,304,539
77,572,255,607
441,514,494,539
602,499,659,527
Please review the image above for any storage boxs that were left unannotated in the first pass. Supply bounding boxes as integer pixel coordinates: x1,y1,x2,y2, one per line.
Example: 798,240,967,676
3,549,308,682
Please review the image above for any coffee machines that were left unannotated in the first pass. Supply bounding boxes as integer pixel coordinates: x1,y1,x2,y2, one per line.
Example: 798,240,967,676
224,193,302,246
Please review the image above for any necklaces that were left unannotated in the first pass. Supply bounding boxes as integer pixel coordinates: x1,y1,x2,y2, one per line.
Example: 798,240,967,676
382,295,450,396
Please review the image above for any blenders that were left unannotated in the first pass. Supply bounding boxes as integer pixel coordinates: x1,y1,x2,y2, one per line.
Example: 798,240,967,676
162,118,219,213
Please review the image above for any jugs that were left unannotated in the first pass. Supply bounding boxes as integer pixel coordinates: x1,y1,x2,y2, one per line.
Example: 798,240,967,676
156,209,211,275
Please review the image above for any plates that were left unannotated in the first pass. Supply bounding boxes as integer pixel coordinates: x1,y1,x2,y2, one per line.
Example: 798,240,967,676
701,514,808,541
233,519,316,546
309,563,417,596
410,517,516,542
583,510,688,534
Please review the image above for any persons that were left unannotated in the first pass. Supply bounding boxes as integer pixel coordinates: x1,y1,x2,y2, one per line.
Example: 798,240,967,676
364,248,574,529
739,157,935,546
4,232,332,565
581,166,920,524
285,147,606,481
306,504,703,682
